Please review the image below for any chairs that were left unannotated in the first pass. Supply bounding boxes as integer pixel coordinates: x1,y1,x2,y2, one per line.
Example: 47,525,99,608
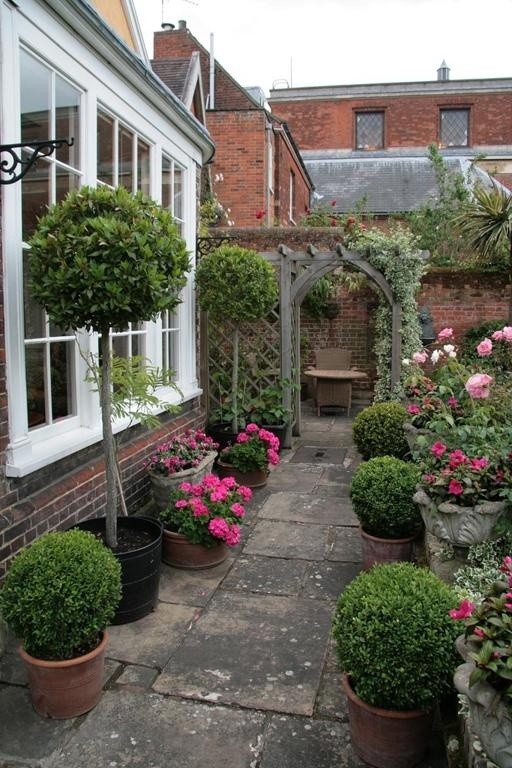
311,339,356,399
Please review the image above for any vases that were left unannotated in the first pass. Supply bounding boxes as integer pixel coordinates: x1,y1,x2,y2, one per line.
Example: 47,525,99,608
157,521,241,572
142,454,231,496
414,492,512,567
443,632,512,760
397,424,485,461
224,452,281,489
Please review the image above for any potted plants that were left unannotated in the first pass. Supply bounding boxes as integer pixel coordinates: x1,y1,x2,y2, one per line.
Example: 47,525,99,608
201,248,290,460
349,453,419,565
352,397,414,468
337,553,462,761
31,182,193,626
1,529,126,725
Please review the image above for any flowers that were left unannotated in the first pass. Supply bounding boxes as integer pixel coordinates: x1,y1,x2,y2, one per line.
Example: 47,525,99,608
124,426,227,476
421,439,512,504
234,419,289,473
387,322,512,424
154,475,259,543
447,542,510,686
253,193,373,230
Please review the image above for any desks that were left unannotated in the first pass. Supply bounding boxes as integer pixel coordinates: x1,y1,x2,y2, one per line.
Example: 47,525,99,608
305,365,369,424
